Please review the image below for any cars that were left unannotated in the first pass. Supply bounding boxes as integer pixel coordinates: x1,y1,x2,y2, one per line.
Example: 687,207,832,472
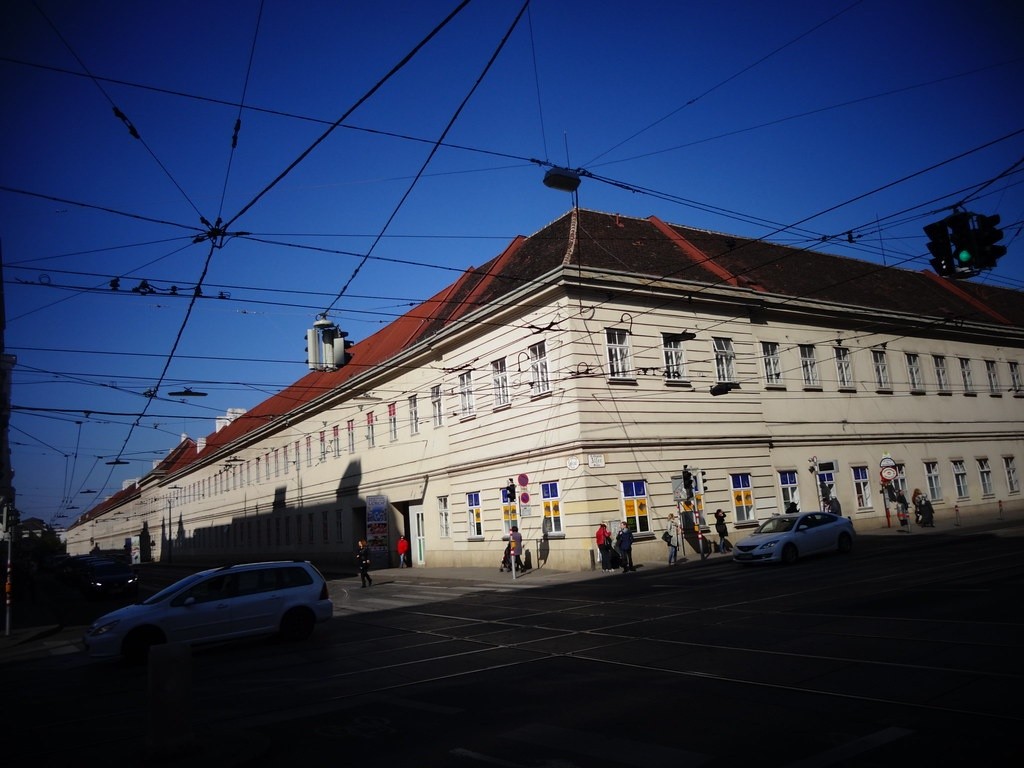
81,558,333,670
732,511,856,566
83,560,136,603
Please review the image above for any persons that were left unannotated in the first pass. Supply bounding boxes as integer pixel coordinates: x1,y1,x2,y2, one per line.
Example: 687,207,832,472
897,490,910,527
822,497,842,517
715,509,731,554
912,489,936,528
397,535,409,568
616,522,636,573
596,524,615,573
508,526,526,573
357,539,372,588
785,502,800,514
666,514,679,568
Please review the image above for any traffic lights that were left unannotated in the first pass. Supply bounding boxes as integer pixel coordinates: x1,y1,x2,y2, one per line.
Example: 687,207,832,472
506,484,516,500
697,470,708,495
944,214,983,280
976,215,1008,271
682,471,694,489
923,220,955,277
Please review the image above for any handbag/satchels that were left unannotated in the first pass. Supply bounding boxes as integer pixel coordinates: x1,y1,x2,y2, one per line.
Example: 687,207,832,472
662,532,672,543
616,537,622,546
605,537,612,549
